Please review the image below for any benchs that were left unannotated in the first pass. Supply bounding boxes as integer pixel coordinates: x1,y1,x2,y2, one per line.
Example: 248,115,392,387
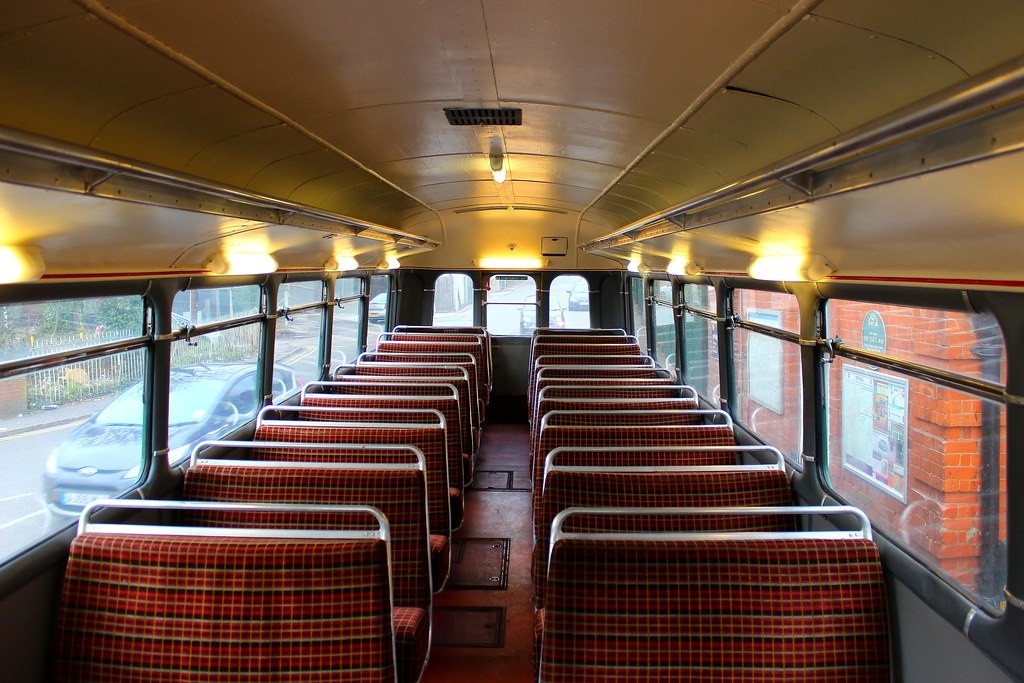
527,327,901,683
49,325,493,683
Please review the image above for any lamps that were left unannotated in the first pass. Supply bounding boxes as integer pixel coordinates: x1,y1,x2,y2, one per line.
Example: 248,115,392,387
0,245,47,284
489,155,505,184
472,257,551,268
325,256,359,270
665,259,702,275
745,256,836,281
624,261,648,272
379,261,400,269
203,252,279,274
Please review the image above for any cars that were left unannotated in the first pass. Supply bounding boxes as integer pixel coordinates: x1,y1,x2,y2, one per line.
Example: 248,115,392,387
517,294,567,334
39,359,303,522
369,293,388,324
566,278,589,312
